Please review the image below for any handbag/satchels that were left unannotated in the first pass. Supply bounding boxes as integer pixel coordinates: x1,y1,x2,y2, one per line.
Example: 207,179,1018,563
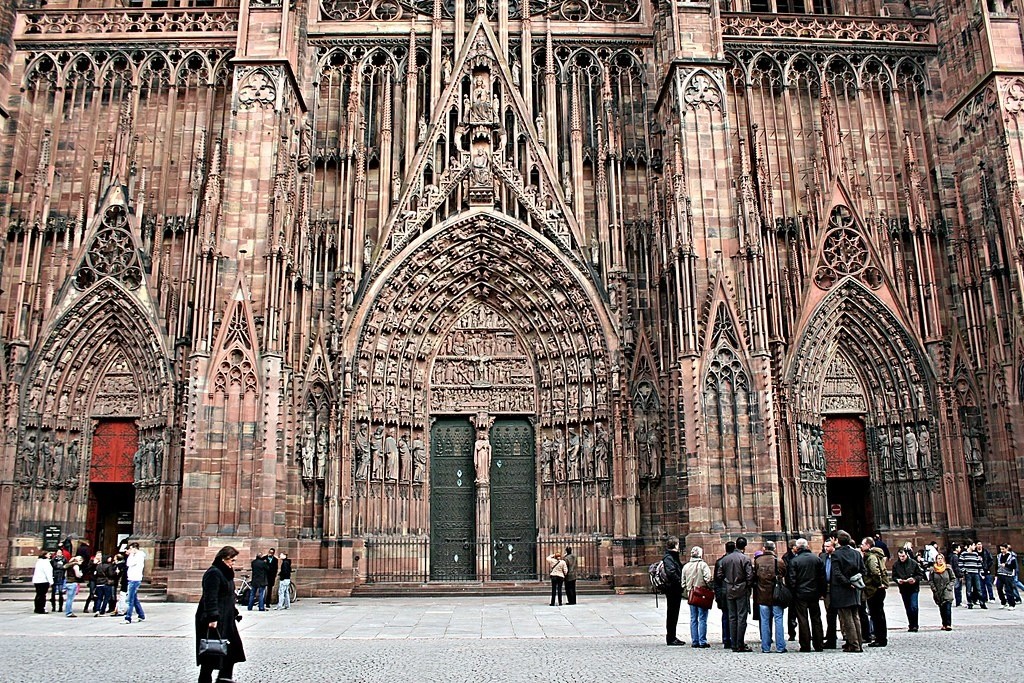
107,580,114,584
772,582,792,606
199,627,228,656
688,585,715,609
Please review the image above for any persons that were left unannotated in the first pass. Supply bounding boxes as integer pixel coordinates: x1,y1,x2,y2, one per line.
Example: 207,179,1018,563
76,539,91,582
546,553,568,606
62,556,85,617
132,434,164,488
512,55,617,309
301,424,328,479
961,421,982,461
63,538,73,554
20,434,79,490
195,545,247,683
818,530,891,652
120,542,146,624
473,146,489,187
915,541,939,581
50,542,72,612
354,423,425,486
247,551,269,611
463,75,499,122
713,537,754,652
271,550,292,611
753,540,789,653
929,553,956,631
681,545,715,648
635,420,663,479
32,549,54,614
344,53,452,310
782,538,828,652
877,425,930,469
540,422,609,482
83,550,128,617
947,539,995,609
561,545,578,604
262,548,278,608
892,547,923,632
796,423,825,475
898,541,920,560
996,542,1024,610
662,535,686,645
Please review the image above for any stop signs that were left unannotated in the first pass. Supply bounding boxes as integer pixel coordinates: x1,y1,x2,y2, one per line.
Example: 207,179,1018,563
831,504,842,517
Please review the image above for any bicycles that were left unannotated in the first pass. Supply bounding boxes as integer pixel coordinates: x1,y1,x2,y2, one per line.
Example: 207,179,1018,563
234,574,265,606
277,570,297,604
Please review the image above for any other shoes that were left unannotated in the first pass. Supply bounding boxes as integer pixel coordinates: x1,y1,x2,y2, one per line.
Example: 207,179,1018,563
135,618,143,622
40,610,48,613
550,604,554,606
120,620,129,624
68,614,76,617
946,626,952,630
956,598,1015,611
83,608,115,617
941,626,946,630
667,636,886,654
566,603,573,604
559,604,562,605
908,627,918,632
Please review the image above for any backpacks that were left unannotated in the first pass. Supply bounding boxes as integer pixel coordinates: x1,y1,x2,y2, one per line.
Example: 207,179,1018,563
648,554,670,590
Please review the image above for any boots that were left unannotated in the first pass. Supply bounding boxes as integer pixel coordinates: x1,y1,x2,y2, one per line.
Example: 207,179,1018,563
58,597,63,612
50,599,55,612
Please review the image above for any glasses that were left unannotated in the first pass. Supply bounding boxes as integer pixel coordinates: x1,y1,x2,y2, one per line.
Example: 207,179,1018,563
230,558,236,562
